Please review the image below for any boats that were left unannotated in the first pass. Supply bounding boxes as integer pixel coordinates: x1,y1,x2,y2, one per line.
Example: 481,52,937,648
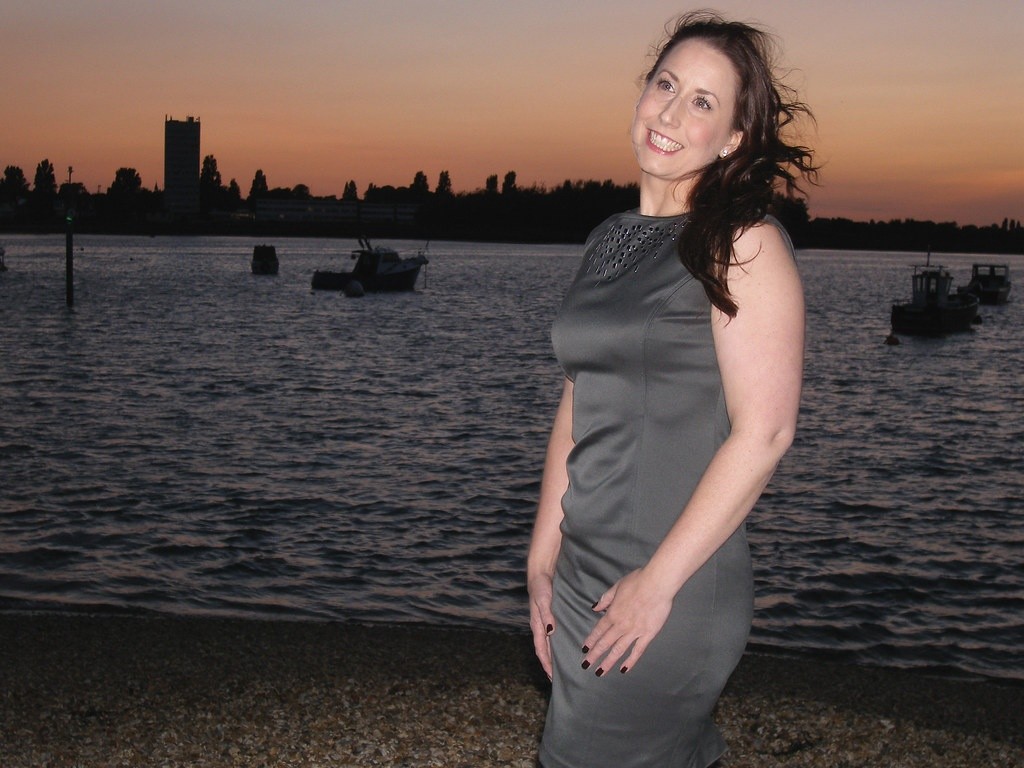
309,246,431,293
887,263,980,340
250,244,280,277
955,261,1012,305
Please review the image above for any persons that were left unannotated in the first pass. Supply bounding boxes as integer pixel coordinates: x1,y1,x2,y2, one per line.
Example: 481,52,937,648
524,9,804,766
